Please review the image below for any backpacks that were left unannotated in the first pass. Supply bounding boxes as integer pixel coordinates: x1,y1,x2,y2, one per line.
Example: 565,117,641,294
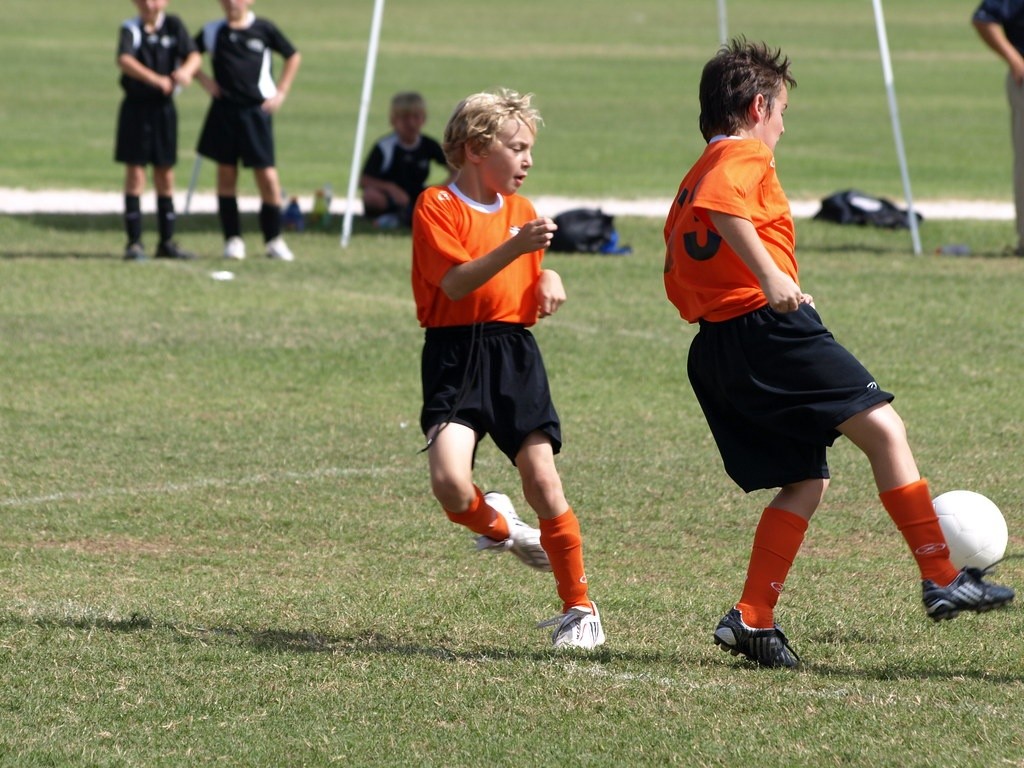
553,208,614,253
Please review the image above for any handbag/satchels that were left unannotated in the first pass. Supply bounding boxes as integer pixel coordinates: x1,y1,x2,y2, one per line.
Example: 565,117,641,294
816,188,921,228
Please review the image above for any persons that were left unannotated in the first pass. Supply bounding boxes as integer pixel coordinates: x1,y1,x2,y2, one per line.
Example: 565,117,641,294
411,90,606,651
972,0,1024,257
362,91,450,231
114,0,203,262
663,35,1016,666
193,0,300,262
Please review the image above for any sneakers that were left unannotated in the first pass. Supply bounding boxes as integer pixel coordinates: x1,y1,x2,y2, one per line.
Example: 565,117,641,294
155,241,190,259
266,239,292,261
225,237,246,260
714,606,800,668
474,490,553,573
126,245,145,260
537,601,605,650
921,566,1015,622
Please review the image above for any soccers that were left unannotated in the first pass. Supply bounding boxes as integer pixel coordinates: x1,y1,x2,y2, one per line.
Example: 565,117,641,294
932,489,1009,574
